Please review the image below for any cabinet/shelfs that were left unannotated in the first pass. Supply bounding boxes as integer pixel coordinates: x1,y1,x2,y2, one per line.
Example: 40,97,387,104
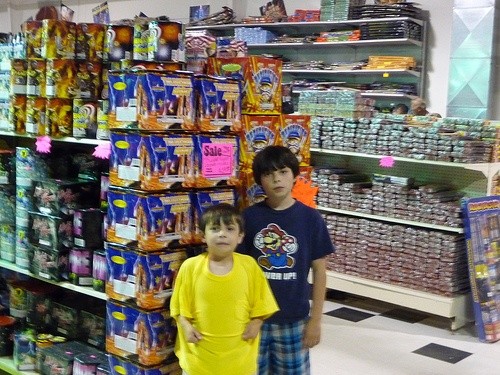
0,17,500,375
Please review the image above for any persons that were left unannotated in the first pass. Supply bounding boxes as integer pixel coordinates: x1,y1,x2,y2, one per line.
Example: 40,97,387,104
234,145,336,374
170,203,281,375
